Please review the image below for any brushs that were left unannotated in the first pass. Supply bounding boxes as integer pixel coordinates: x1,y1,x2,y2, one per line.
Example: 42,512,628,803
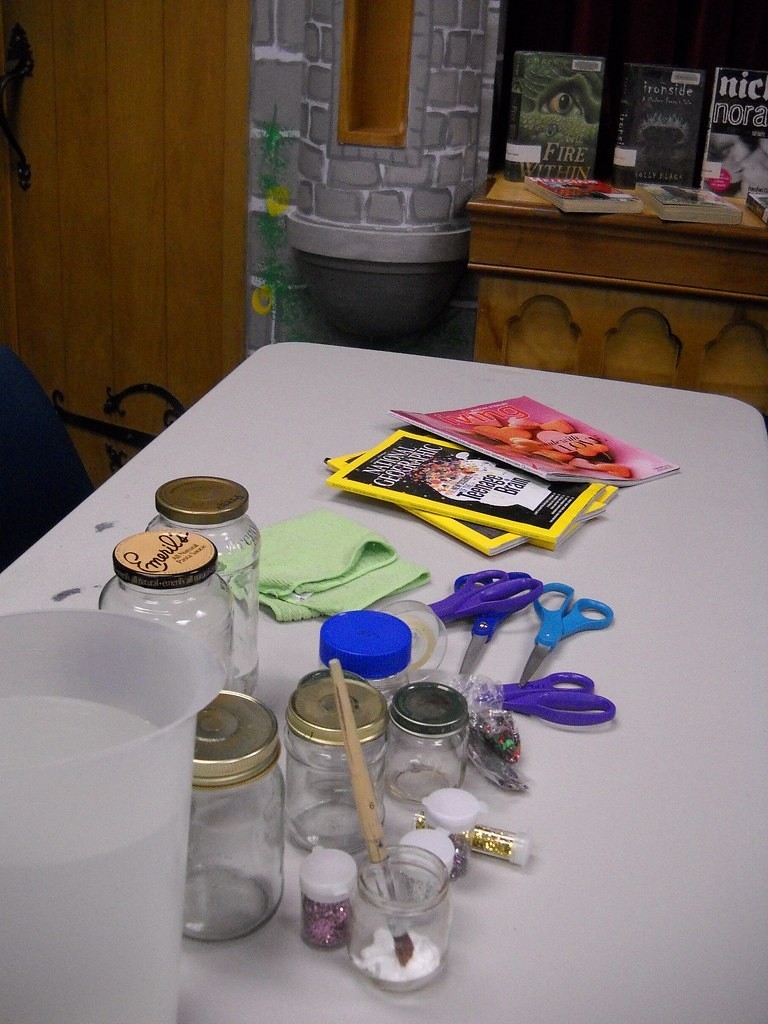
328,657,417,968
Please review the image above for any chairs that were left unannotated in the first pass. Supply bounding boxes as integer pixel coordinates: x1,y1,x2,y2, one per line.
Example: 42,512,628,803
0,341,187,573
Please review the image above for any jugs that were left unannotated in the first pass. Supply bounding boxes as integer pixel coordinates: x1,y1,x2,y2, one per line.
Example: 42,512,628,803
0,608,228,1024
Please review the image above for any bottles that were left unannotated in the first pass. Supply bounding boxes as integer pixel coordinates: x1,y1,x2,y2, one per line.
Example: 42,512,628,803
320,611,411,686
99,530,234,690
399,827,454,882
300,844,355,949
181,688,285,943
145,476,262,696
346,845,452,993
284,677,389,851
422,790,488,879
390,682,470,808
412,810,532,868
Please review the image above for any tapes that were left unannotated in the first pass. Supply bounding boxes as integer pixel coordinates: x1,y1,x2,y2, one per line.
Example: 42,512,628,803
399,614,437,669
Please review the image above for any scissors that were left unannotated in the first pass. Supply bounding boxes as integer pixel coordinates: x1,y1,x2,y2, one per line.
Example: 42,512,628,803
463,672,616,726
426,570,544,676
517,582,613,687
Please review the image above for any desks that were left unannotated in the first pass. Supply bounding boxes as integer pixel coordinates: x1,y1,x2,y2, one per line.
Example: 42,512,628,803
466,170,768,416
0,340,768,1024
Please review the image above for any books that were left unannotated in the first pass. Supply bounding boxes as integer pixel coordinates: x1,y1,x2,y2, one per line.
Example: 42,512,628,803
324,450,608,557
529,485,619,551
388,396,681,487
697,65,768,196
504,49,606,184
746,194,768,225
524,176,644,214
609,60,705,189
324,429,608,544
634,181,744,225
